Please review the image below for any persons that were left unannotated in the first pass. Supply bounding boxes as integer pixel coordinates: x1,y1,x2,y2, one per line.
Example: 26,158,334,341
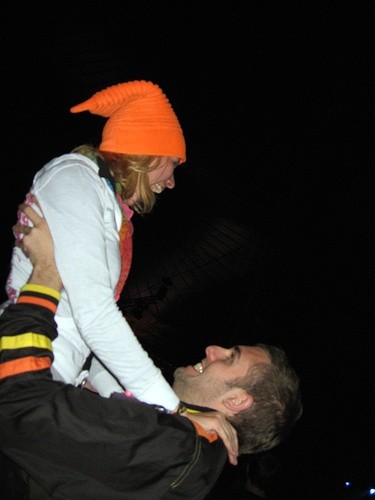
204,452,286,500
1,80,240,467
0,195,302,500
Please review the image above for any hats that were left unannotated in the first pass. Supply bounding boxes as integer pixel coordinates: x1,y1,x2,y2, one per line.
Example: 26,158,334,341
71,81,187,163
